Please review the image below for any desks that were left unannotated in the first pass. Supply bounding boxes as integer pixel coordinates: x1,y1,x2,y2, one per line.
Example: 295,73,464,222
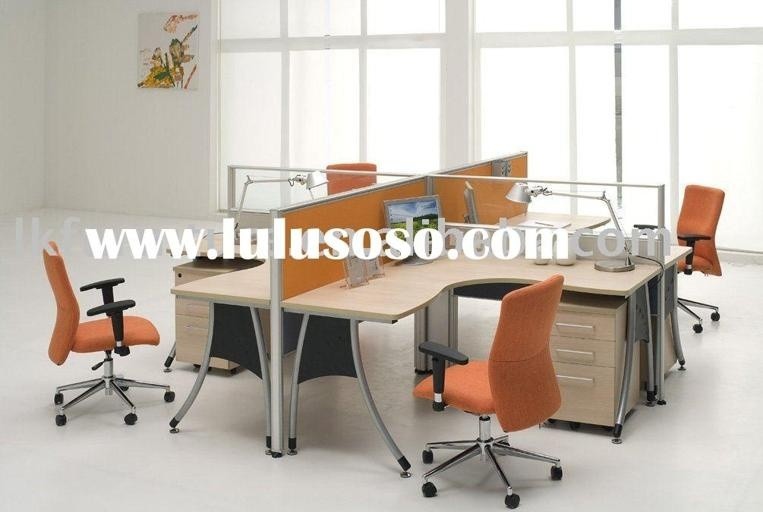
168,233,273,449
488,214,692,373
284,251,659,454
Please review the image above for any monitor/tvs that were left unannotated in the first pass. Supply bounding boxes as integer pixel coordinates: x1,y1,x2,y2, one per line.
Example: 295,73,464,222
382,194,443,267
462,180,479,224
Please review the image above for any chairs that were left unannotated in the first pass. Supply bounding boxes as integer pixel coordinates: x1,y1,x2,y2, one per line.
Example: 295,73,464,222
675,186,725,335
411,274,565,509
326,163,377,196
45,241,176,427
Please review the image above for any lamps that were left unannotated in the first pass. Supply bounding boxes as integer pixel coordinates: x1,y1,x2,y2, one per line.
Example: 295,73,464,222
505,183,637,273
230,169,329,246
